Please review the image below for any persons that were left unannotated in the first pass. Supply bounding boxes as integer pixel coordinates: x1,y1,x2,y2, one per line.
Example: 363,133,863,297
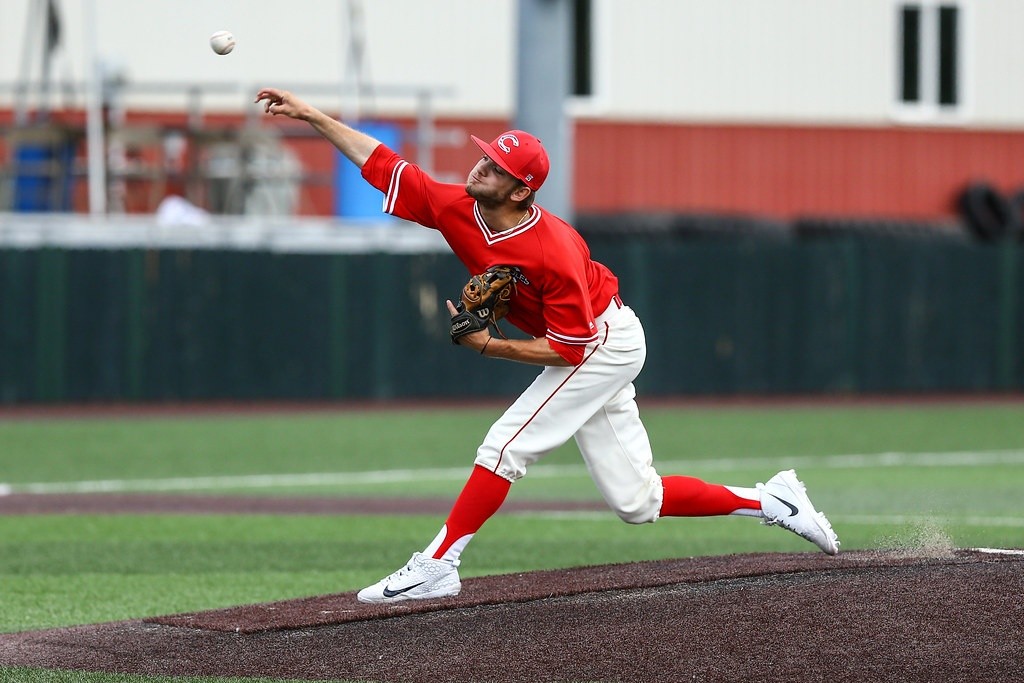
254,84,841,605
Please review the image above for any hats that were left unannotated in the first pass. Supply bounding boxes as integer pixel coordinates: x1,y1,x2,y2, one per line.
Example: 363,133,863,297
470,129,550,191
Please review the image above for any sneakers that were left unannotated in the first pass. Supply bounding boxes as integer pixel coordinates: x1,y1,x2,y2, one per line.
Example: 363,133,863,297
756,468,841,556
357,551,462,604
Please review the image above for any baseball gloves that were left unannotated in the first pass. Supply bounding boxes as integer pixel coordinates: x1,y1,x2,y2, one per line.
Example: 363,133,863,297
449,267,518,346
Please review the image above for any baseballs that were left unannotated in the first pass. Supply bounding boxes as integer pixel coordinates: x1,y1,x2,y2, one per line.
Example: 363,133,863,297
209,30,236,55
